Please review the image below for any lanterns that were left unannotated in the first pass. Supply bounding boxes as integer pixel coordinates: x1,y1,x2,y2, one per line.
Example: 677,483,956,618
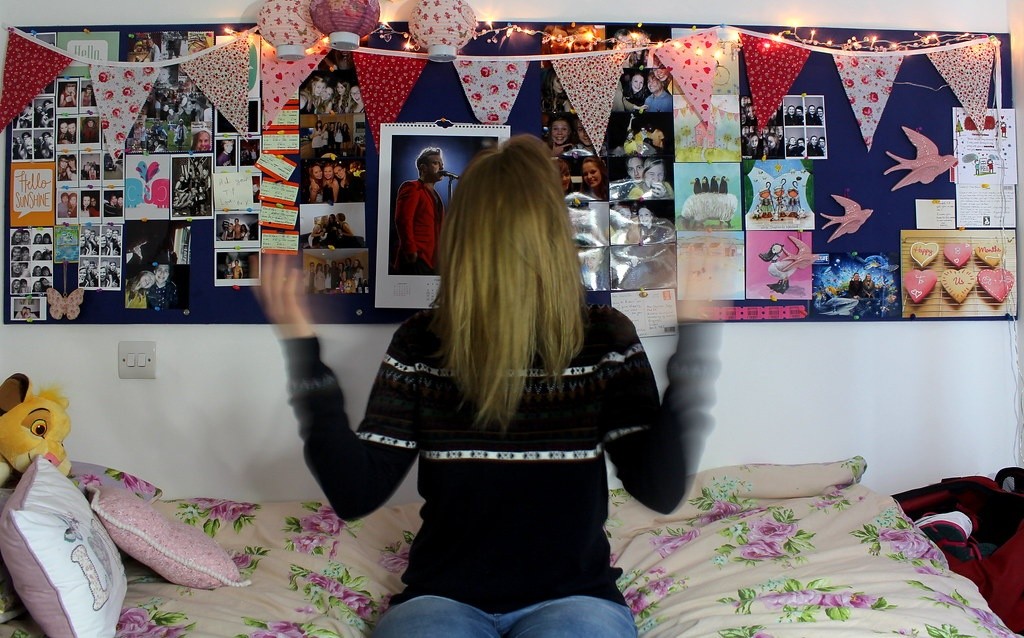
409,0,477,62
257,0,323,61
309,0,381,51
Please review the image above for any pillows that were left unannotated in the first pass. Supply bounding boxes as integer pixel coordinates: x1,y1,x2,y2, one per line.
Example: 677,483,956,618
84,484,252,589
0,454,128,638
0,460,163,624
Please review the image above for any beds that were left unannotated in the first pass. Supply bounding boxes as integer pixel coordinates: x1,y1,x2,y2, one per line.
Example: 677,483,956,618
0,455,1024,638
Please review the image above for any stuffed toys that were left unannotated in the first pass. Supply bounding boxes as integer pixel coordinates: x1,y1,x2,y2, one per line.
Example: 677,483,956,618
0,373,72,486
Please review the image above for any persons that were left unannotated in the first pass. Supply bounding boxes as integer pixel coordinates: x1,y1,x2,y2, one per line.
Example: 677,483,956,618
309,162,364,202
311,212,354,248
623,51,672,112
611,116,675,289
311,120,351,150
741,97,782,155
395,148,445,274
254,136,723,638
123,33,256,309
545,71,609,287
305,258,364,293
300,52,365,113
10,84,122,317
544,26,605,54
849,273,875,299
784,105,826,157
